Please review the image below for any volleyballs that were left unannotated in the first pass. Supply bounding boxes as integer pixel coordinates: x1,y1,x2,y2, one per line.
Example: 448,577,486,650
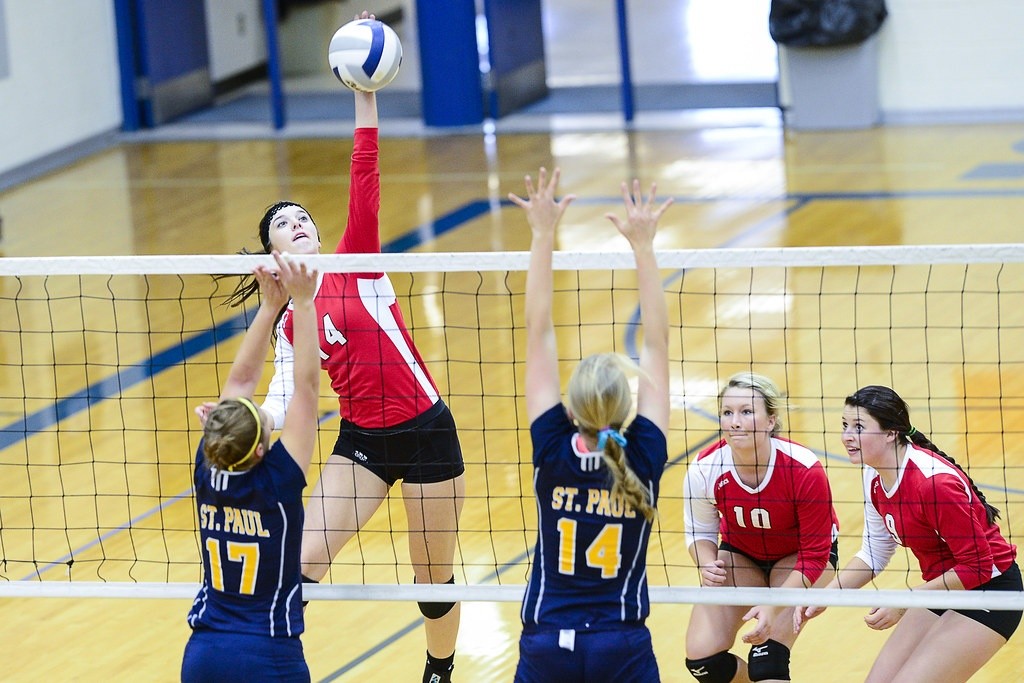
326,18,404,94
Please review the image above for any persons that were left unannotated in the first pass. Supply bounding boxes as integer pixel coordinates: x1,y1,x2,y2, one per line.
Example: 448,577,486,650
507,164,671,683
242,4,469,683
679,369,839,682
177,249,321,683
785,387,1023,682
